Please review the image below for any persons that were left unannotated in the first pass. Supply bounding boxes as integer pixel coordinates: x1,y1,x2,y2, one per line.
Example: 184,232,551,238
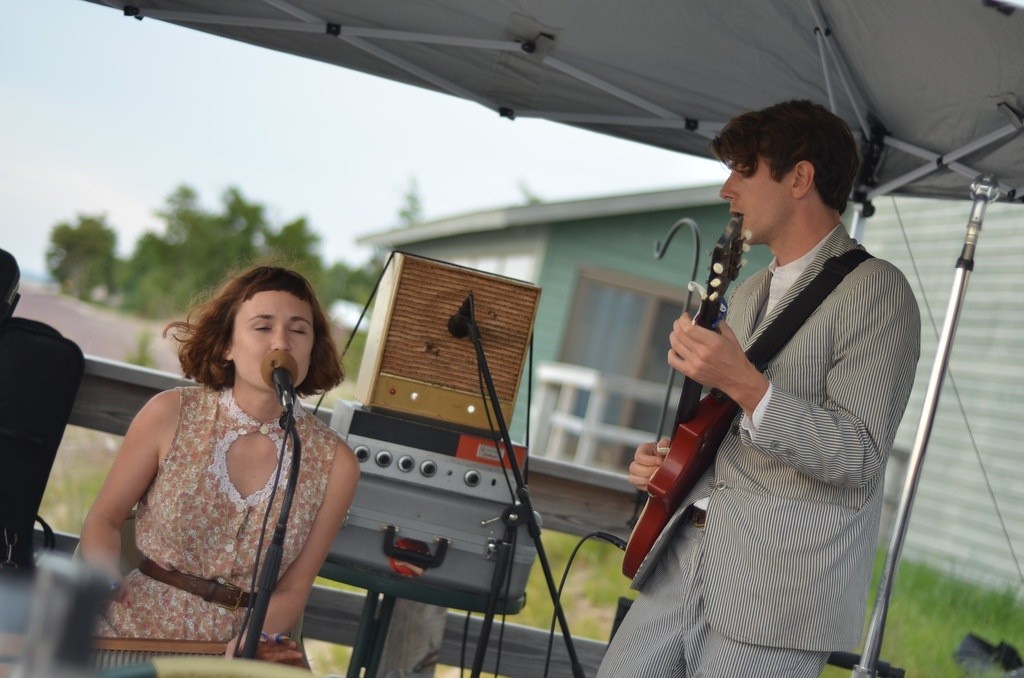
81,265,360,660
595,97,921,678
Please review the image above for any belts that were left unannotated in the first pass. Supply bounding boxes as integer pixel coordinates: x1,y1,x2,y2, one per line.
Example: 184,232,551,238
139,558,259,612
689,508,705,526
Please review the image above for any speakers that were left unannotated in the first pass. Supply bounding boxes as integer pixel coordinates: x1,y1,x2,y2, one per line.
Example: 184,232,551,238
353,251,542,440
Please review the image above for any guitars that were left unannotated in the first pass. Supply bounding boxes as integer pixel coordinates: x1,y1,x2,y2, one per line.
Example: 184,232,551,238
621,211,754,582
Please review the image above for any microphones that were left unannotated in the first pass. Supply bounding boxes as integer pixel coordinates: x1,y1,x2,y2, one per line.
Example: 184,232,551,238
260,350,299,414
449,294,474,339
969,174,1000,202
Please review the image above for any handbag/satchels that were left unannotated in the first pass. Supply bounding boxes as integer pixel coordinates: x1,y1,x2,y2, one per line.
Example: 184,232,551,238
0,507,55,631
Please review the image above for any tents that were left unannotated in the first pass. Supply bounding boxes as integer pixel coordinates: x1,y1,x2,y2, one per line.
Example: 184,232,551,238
80,0,1024,677
